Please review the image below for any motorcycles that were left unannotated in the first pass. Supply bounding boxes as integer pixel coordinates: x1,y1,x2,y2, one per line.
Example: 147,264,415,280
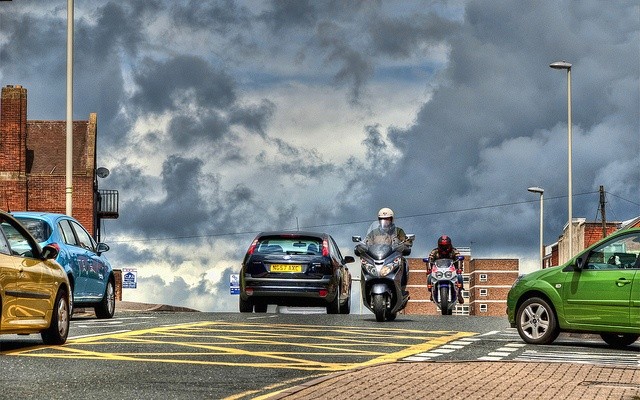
422,255,466,316
353,235,415,322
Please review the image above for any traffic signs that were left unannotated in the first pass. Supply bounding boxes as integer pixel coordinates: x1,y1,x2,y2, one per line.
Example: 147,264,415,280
230,273,240,294
122,268,137,288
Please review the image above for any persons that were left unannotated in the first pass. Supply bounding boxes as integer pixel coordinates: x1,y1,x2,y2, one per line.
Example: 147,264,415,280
425,234,466,305
354,207,411,296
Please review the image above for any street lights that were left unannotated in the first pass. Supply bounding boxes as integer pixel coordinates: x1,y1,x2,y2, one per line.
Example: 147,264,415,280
529,187,545,270
550,61,572,261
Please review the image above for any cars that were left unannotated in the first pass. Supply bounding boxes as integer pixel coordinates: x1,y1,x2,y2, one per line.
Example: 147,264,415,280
239,231,354,314
9,213,114,318
507,229,640,348
0,211,72,344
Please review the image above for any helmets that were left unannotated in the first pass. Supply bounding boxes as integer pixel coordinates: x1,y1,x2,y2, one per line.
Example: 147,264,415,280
377,208,394,224
438,236,452,255
